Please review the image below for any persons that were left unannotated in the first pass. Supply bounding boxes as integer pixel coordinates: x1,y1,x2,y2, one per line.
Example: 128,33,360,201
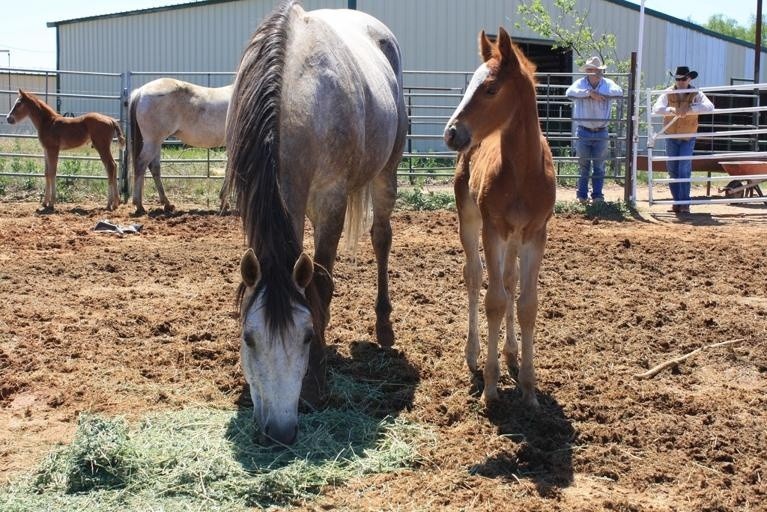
652,66,714,213
566,57,623,203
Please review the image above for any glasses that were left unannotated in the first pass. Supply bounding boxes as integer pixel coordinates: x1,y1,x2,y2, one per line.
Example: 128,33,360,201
676,77,688,81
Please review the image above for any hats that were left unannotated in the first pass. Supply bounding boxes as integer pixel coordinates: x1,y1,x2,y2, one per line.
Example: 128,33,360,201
669,67,698,79
579,57,607,72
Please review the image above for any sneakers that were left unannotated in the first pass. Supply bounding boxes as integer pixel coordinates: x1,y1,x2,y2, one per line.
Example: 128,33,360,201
576,197,604,203
667,204,690,213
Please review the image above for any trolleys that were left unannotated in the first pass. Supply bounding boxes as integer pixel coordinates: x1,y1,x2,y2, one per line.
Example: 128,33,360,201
718,161,767,206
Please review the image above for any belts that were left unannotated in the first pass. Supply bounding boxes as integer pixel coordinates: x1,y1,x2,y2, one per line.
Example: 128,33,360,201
583,127,604,131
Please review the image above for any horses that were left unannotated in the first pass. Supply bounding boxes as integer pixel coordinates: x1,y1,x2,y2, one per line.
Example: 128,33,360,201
6,87,130,215
220,0,411,453
442,25,559,410
116,75,236,216
